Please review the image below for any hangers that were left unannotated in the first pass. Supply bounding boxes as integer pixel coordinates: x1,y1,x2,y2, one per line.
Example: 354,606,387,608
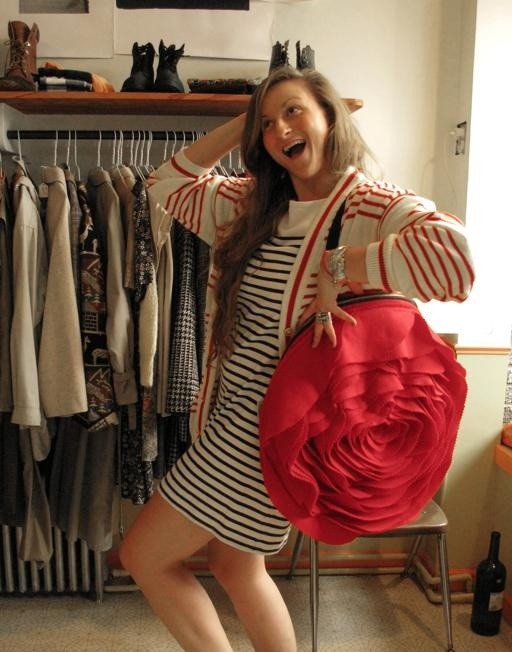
0,126,254,187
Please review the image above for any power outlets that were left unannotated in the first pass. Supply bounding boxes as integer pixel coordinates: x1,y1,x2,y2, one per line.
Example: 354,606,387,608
455,120,467,155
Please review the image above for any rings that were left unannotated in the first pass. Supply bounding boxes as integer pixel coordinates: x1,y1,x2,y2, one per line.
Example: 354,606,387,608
318,312,329,323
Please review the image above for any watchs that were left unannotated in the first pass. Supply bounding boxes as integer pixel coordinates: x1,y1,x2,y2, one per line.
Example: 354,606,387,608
328,246,347,281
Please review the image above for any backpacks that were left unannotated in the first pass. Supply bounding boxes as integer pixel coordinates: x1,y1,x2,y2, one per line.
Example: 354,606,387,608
258,197,468,545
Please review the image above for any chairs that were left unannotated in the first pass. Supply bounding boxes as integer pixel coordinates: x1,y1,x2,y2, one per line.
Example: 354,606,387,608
288,331,460,651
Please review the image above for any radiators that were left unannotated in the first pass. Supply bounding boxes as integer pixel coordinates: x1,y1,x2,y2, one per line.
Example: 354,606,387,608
1,524,109,602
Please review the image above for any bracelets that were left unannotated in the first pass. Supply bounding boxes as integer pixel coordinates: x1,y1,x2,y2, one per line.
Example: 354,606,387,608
118,65,475,652
320,251,331,280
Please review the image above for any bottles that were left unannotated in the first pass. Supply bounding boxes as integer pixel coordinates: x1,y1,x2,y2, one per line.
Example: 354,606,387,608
470,531,507,636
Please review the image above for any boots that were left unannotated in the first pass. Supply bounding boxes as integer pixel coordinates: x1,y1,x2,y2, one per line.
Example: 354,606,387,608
0,21,40,91
155,39,186,92
120,42,155,92
296,40,316,71
269,40,291,74
